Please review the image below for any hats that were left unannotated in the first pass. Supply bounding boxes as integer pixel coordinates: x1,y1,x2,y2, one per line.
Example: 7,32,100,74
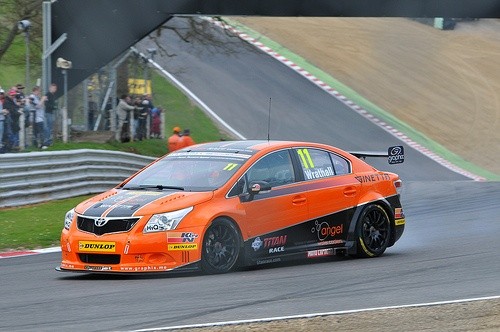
8,91,16,94
16,84,24,89
0,89,5,95
173,127,180,133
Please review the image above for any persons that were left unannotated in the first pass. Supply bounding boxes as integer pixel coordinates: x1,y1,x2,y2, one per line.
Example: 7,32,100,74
176,129,195,149
167,126,182,151
0,83,60,154
81,88,166,142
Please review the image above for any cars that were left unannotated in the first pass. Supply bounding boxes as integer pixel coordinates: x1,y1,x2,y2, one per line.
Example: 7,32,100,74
55,139,407,276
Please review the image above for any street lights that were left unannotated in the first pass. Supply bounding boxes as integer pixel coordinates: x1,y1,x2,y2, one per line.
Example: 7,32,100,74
17,19,32,96
